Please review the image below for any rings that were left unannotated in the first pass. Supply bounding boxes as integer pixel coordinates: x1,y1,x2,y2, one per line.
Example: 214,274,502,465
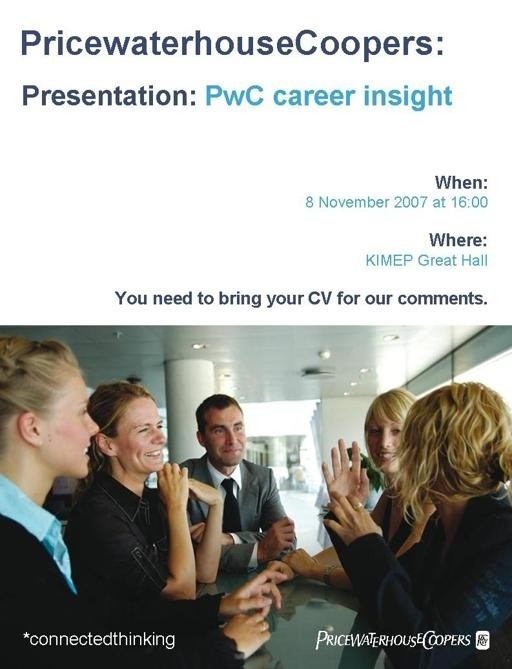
353,502,362,509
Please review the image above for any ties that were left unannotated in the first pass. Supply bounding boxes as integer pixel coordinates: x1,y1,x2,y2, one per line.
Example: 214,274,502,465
221,478,243,535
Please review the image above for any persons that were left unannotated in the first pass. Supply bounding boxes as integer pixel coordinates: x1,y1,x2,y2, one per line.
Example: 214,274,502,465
179,395,298,575
0,337,288,669
63,381,225,601
322,382,512,669
281,389,437,592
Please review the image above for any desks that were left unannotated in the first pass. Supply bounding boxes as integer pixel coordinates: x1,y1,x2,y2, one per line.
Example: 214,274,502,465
190,561,403,669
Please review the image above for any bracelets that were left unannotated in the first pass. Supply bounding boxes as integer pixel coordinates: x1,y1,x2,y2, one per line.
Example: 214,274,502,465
323,565,335,584
